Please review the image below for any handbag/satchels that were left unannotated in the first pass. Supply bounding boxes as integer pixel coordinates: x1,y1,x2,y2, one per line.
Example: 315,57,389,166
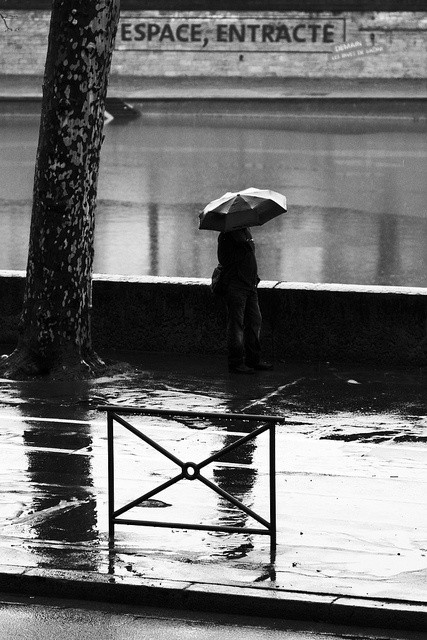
209,265,225,293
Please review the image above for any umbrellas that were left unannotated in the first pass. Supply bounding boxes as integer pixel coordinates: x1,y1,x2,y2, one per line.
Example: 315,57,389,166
198,186,288,232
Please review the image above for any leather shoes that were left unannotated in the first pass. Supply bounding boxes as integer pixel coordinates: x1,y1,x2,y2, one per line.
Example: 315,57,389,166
250,360,272,371
228,364,257,374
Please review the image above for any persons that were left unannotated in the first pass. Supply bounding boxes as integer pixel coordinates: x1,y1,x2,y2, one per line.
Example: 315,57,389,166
208,228,274,374
